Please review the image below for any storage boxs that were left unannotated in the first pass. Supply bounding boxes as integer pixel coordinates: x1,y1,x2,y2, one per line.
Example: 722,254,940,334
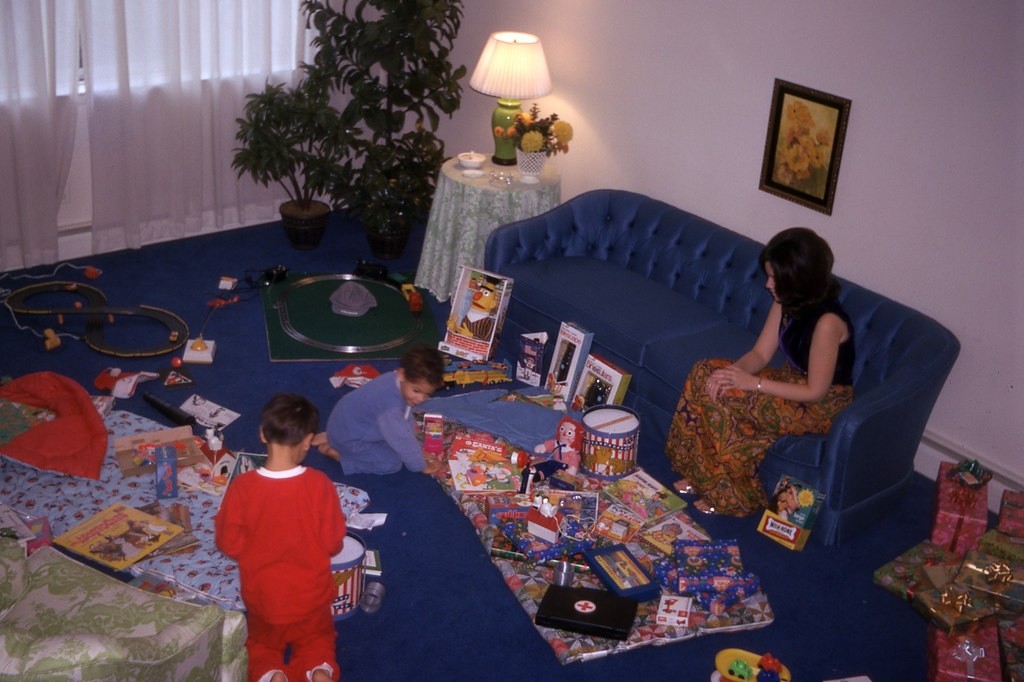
488,471,828,642
870,461,1024,682
437,264,513,364
544,322,594,402
569,352,632,414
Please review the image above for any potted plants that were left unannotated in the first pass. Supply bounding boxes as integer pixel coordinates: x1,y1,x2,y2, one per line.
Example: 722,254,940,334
297,0,468,260
232,78,352,241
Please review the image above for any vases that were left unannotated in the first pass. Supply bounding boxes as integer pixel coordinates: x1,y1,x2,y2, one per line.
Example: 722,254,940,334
514,146,546,188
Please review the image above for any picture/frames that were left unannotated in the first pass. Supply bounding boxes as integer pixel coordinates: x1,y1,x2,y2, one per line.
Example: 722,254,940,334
756,78,851,215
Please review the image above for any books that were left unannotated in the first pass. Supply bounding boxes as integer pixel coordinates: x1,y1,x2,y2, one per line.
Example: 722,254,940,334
542,469,714,558
448,434,521,495
115,425,205,476
53,502,200,572
0,503,52,558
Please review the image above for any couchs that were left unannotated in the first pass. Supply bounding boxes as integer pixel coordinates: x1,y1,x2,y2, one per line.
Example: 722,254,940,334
0,535,250,682
485,188,965,547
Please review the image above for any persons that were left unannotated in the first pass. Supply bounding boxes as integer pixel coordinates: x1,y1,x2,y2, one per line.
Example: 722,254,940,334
310,342,444,475
213,394,347,682
521,417,584,481
664,226,857,517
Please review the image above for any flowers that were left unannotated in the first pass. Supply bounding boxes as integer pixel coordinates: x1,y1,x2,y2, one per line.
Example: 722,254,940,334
492,105,576,161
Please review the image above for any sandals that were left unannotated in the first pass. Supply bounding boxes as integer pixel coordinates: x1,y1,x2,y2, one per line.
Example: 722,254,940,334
693,498,749,517
674,479,697,493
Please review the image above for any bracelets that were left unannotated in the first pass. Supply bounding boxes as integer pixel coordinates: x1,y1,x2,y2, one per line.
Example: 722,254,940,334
757,378,762,390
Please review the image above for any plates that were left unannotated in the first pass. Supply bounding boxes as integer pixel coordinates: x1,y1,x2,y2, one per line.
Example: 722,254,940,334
463,171,485,177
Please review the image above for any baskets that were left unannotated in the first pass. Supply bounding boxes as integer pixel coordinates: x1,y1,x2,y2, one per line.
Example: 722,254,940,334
514,145,547,175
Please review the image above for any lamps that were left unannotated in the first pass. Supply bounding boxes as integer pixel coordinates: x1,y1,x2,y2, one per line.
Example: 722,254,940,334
473,26,553,165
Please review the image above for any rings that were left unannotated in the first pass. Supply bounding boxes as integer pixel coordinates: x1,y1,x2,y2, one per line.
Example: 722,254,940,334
709,384,711,387
706,383,708,385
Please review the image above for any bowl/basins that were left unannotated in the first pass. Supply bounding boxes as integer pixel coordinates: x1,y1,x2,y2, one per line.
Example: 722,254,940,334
458,151,486,169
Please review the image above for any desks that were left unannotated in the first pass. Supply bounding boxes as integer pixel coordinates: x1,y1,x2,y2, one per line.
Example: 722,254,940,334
413,159,560,313
